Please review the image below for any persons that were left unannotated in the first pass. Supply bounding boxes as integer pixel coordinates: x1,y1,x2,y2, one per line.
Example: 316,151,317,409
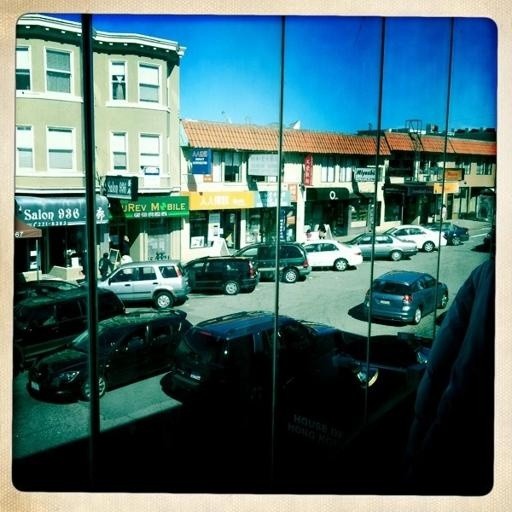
318,222,326,239
407,258,493,493
99,235,134,281
226,232,233,249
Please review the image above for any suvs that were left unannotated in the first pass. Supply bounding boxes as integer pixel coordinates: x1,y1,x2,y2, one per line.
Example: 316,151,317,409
231,241,313,283
95,259,192,309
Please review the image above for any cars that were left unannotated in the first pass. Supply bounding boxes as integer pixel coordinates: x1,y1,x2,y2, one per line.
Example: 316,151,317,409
301,239,363,271
363,270,448,324
27,306,193,403
170,311,339,398
384,224,448,251
14,279,126,372
345,232,418,262
422,221,469,245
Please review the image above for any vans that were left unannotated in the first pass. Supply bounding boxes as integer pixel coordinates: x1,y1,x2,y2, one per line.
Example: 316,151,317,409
183,256,258,295
